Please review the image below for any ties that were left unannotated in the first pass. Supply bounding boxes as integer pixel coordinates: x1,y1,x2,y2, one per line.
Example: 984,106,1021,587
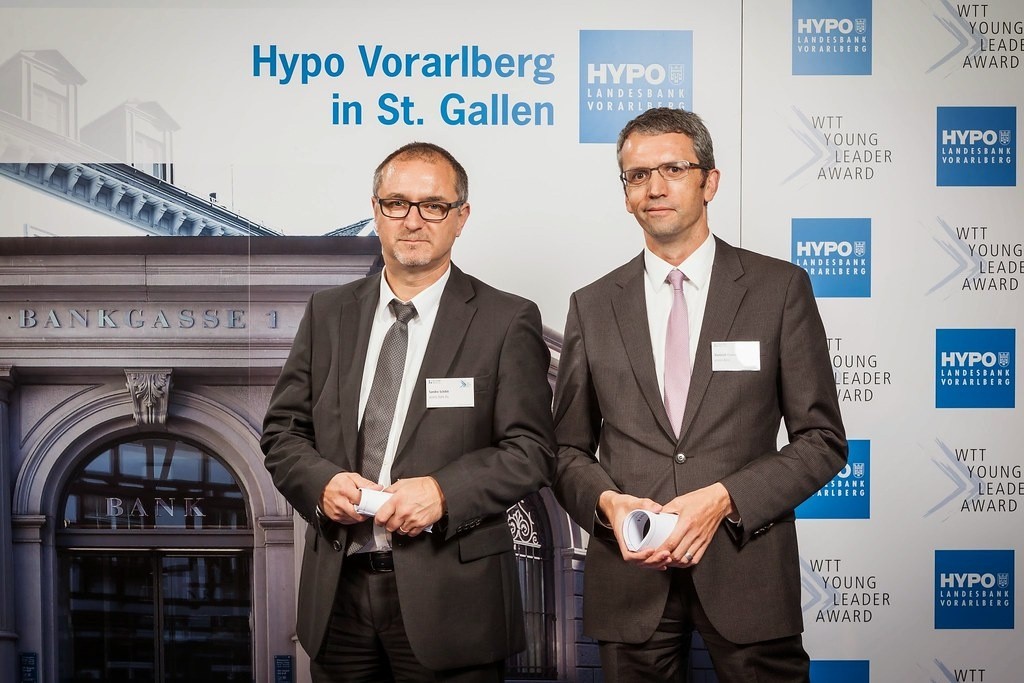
665,270,691,440
344,298,419,557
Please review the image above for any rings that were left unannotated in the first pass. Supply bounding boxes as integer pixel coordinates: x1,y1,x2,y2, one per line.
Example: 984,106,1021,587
684,552,693,561
399,527,409,533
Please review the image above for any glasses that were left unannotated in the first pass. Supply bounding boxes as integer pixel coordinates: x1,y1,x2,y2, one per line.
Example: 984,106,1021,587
376,195,464,220
619,160,711,198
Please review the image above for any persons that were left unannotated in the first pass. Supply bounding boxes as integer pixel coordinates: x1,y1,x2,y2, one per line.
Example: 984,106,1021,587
552,107,849,683
260,143,551,683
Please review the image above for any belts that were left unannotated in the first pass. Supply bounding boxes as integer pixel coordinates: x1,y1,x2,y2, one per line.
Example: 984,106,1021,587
345,552,394,573
